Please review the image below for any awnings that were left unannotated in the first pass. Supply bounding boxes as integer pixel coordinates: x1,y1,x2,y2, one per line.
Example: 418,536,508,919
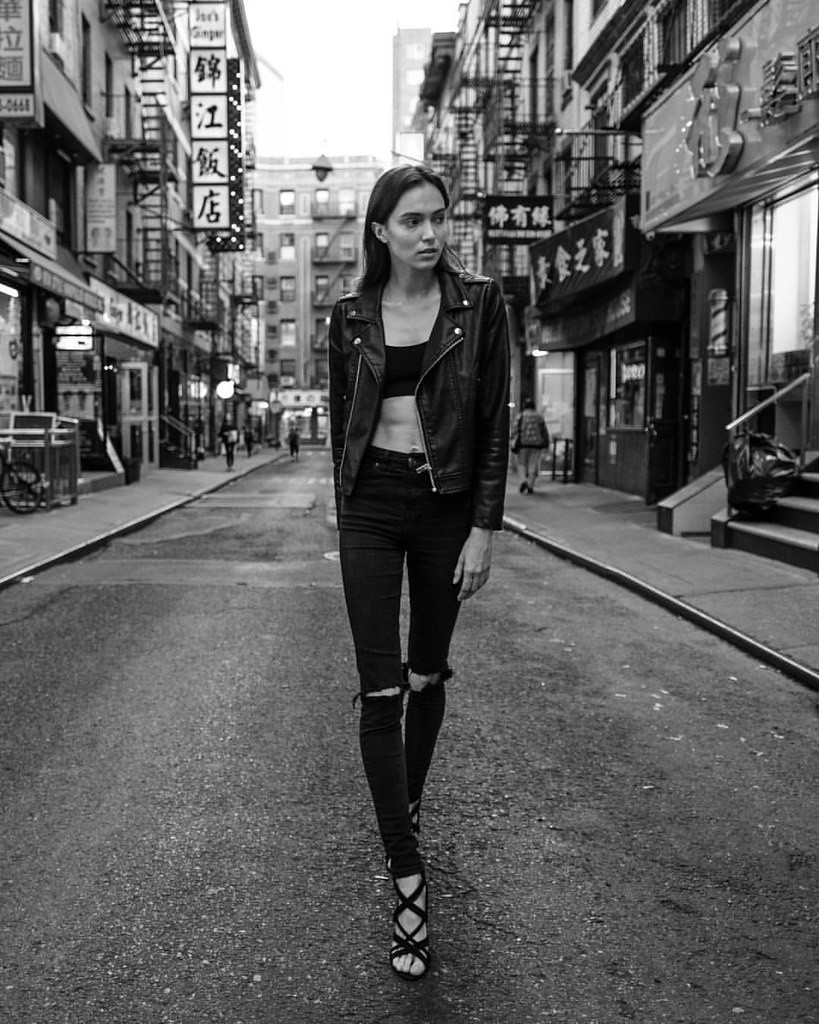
0,233,105,314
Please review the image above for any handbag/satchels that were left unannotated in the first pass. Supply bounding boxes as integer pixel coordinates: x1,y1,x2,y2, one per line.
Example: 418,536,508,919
511,413,523,453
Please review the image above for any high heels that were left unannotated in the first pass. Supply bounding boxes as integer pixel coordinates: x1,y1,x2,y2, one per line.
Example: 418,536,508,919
386,798,422,871
390,872,430,980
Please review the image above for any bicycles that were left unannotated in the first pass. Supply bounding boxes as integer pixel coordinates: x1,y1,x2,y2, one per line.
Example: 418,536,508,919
0,447,42,513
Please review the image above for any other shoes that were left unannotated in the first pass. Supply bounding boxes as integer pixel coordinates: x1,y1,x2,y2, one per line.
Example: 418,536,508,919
520,482,528,493
527,487,533,495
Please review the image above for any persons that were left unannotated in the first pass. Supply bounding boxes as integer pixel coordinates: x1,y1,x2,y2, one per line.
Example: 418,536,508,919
288,428,299,461
329,165,514,986
509,398,550,494
218,411,240,472
242,426,260,457
